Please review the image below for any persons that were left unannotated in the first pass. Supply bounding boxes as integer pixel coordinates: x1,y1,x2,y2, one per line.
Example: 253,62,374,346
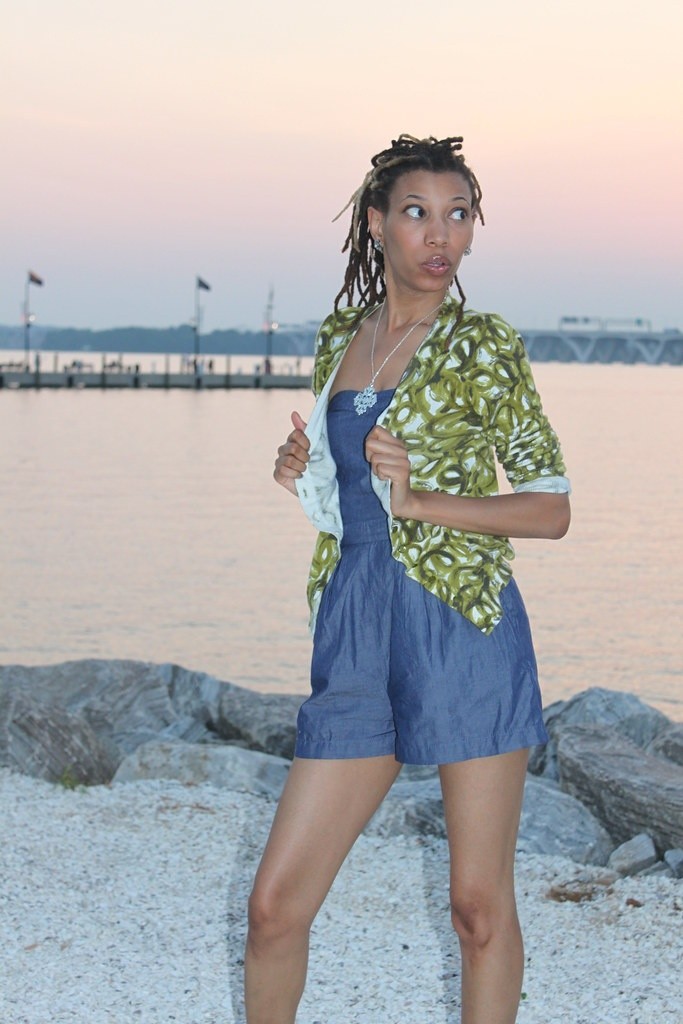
245,133,571,1024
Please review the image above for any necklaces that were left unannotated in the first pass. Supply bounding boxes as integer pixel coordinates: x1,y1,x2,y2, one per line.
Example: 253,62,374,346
354,295,444,416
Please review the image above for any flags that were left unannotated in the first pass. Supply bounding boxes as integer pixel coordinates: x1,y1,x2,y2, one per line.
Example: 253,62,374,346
29,272,42,285
194,277,210,290
